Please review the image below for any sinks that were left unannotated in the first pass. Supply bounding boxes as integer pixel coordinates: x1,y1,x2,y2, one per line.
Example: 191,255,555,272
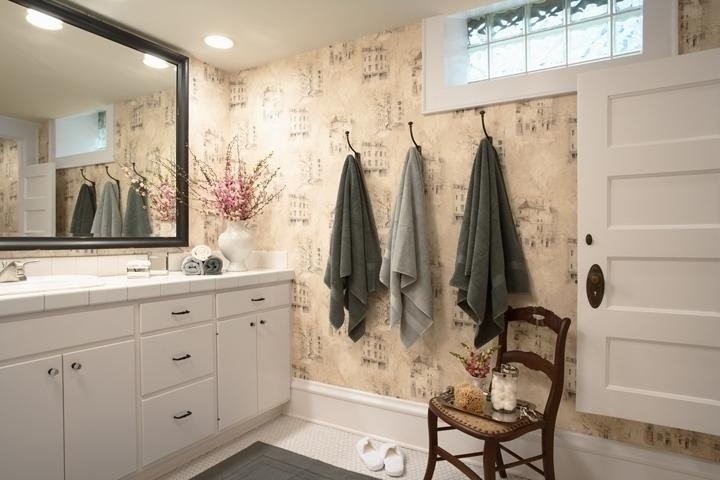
1,273,104,294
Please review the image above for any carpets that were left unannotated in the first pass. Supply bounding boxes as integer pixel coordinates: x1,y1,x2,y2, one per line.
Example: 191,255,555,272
188,440,382,480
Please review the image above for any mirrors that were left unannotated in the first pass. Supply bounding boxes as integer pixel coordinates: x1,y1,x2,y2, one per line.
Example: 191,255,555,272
1,1,191,252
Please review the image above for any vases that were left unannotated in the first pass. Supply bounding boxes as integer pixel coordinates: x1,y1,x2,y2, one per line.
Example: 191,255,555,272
474,377,486,392
218,221,254,273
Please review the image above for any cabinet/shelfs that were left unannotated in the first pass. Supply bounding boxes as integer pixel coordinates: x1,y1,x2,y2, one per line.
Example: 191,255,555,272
139,292,215,468
1,339,138,479
217,280,296,436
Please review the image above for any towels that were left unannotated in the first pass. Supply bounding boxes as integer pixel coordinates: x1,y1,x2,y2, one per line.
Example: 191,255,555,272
121,183,153,237
379,145,437,350
447,137,534,353
179,245,224,276
323,150,383,342
90,182,123,236
69,183,96,236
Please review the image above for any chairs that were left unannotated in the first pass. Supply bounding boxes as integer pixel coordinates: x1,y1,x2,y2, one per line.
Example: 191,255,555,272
425,303,574,480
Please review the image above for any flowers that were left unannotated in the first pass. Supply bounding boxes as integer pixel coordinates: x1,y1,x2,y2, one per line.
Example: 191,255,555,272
113,144,176,225
448,337,503,378
156,137,296,222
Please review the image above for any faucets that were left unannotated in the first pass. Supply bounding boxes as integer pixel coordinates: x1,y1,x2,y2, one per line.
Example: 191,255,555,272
0,260,24,283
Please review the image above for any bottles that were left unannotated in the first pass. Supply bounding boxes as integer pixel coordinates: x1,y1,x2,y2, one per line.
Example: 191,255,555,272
490,362,521,413
